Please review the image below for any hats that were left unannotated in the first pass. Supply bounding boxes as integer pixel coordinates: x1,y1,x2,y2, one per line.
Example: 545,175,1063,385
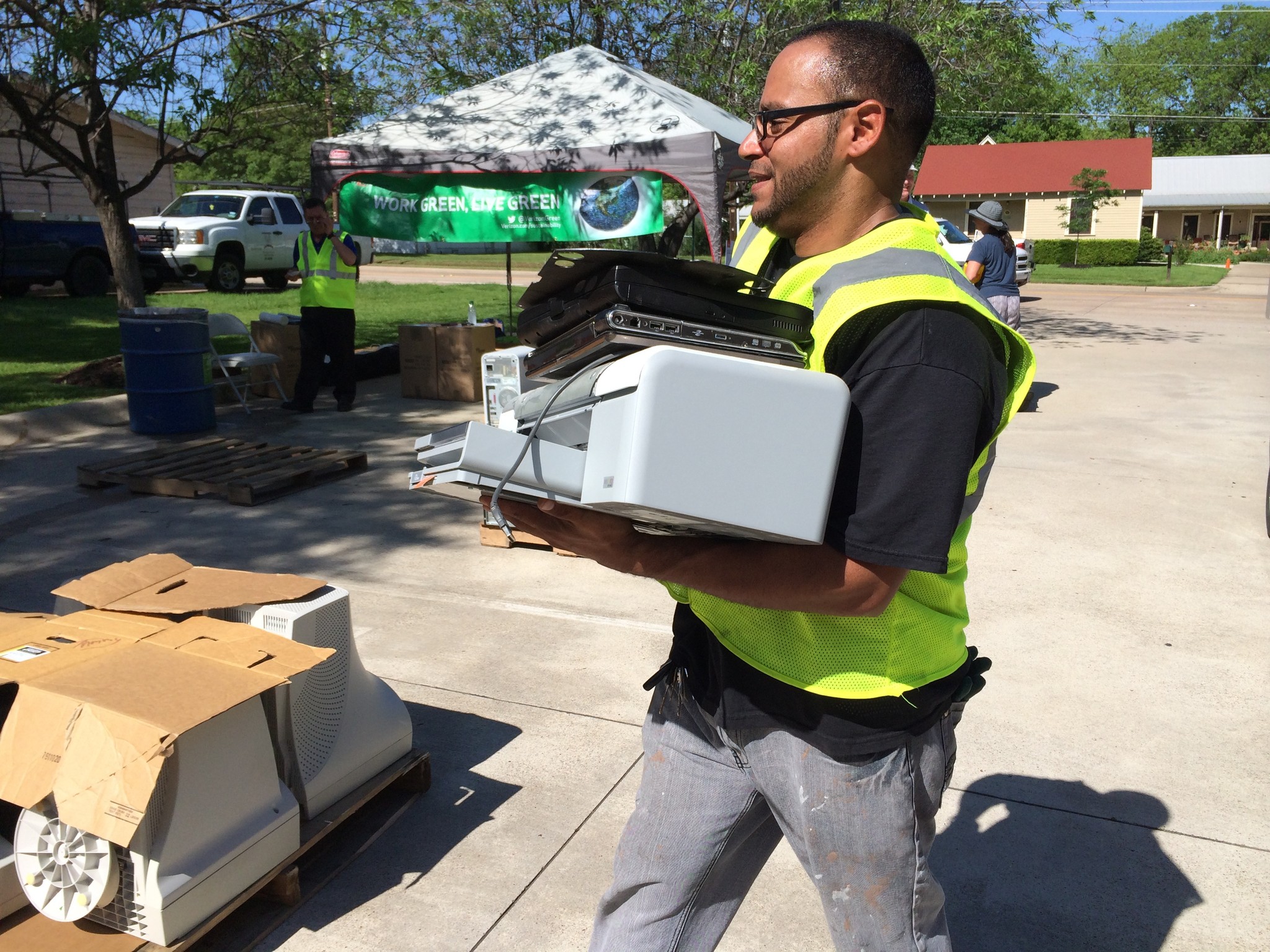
965,200,1009,229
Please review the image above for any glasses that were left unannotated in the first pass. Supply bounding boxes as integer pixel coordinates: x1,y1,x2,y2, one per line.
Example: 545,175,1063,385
306,216,327,223
752,100,894,143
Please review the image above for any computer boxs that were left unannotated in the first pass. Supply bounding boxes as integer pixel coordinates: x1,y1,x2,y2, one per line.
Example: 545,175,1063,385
482,345,548,428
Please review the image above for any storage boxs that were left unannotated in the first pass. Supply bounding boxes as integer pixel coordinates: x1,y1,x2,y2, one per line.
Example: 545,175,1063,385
249,320,300,399
398,322,496,403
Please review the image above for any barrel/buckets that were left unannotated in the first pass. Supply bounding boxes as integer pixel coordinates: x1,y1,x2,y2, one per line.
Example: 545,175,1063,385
117,307,216,435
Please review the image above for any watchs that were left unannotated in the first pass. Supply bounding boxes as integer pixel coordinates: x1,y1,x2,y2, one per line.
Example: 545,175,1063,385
328,232,336,239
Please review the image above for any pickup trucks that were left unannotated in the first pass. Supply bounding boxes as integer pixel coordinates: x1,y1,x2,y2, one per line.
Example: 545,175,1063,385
128,190,376,294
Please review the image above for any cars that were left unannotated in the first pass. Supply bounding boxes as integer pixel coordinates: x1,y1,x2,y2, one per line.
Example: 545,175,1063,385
933,217,1031,288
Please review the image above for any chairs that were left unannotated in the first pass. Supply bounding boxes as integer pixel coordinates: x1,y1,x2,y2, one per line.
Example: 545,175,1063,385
208,313,290,416
1203,235,1210,246
1228,235,1239,249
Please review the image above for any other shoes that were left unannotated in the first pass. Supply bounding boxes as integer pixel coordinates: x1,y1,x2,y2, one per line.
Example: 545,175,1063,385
336,401,352,412
279,399,313,411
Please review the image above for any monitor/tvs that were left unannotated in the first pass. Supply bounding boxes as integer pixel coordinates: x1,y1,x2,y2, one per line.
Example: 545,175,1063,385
13,583,414,947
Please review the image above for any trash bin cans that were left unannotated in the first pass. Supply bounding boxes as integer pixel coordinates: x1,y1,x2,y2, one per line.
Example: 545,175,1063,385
119,306,219,435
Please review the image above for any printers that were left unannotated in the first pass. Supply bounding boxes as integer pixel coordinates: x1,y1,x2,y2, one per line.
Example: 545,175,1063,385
407,344,852,544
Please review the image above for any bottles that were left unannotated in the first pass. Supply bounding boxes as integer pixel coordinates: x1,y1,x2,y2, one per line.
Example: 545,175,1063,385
467,300,477,326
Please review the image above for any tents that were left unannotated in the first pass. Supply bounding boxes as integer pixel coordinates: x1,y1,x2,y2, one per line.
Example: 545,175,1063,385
310,44,768,338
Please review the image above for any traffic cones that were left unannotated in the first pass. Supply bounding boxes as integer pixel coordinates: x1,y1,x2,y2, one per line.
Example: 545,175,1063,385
1225,258,1231,269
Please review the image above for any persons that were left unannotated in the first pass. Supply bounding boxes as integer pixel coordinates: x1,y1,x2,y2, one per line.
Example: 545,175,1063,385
477,20,1036,952
286,197,358,413
965,201,1022,335
899,164,930,215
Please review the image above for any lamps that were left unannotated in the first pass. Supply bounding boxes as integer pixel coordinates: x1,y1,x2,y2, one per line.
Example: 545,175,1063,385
1006,200,1009,205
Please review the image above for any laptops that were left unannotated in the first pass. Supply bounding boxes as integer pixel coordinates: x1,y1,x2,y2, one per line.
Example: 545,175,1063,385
516,265,815,351
523,304,809,383
